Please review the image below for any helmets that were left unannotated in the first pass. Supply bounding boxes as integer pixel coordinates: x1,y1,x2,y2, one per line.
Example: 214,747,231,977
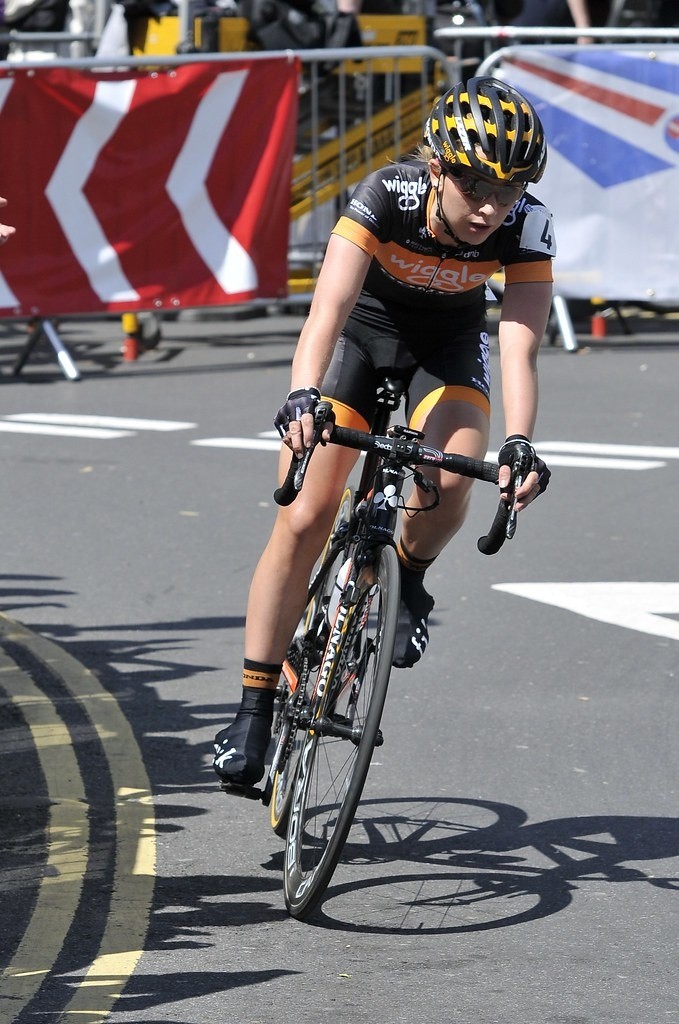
422,77,548,184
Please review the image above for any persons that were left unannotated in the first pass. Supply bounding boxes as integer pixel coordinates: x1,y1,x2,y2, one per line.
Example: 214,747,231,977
215,76,557,790
243,0,613,153
0,0,70,61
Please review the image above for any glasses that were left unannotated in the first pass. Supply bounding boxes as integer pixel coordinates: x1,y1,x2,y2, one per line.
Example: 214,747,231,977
434,155,528,207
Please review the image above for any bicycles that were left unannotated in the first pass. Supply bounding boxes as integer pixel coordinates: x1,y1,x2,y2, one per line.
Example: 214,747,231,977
219,401,540,919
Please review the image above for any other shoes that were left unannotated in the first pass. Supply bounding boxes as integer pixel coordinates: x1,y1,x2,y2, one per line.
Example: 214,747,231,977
391,560,436,667
213,685,275,783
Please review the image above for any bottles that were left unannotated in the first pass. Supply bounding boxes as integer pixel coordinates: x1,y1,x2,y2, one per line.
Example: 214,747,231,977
327,557,352,628
